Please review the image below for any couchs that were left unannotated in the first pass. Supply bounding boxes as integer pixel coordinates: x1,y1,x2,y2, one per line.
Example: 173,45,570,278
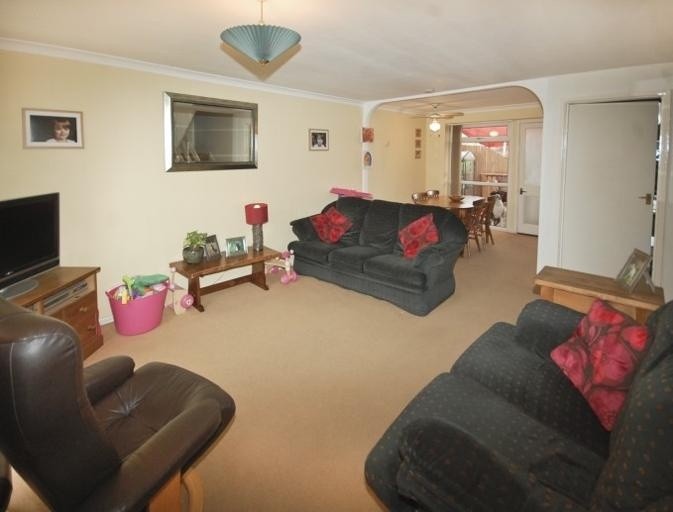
287,196,469,317
365,299,673,511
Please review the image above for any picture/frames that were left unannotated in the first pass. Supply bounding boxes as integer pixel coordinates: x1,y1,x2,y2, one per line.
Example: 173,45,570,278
308,129,330,151
163,91,259,172
615,248,655,295
21,107,85,150
226,236,247,258
205,234,222,262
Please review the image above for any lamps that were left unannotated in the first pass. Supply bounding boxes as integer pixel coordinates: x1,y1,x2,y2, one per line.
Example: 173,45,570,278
220,0,301,66
245,203,268,252
415,103,454,137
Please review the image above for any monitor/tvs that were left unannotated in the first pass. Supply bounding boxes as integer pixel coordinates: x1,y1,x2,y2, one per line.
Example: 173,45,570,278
0,192,60,299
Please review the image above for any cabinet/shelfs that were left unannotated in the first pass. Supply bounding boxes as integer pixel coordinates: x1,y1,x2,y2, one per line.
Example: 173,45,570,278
0,267,105,362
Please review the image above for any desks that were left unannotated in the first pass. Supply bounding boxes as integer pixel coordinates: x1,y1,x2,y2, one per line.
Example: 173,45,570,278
533,265,666,325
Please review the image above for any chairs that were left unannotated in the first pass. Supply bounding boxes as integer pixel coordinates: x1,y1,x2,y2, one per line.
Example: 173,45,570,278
0,296,236,512
487,195,498,245
426,190,440,199
461,199,486,256
411,192,432,203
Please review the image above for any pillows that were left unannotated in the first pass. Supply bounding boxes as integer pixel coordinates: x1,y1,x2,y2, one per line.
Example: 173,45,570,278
398,213,440,257
550,299,649,432
308,205,354,244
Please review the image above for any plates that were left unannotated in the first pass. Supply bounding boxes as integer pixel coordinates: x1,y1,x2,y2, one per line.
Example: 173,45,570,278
449,195,464,202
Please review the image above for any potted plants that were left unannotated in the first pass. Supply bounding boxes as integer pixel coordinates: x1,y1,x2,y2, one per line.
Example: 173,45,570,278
182,231,208,264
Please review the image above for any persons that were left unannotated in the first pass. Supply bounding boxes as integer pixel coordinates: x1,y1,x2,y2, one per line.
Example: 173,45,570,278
313,134,326,148
625,261,637,286
46,118,76,143
